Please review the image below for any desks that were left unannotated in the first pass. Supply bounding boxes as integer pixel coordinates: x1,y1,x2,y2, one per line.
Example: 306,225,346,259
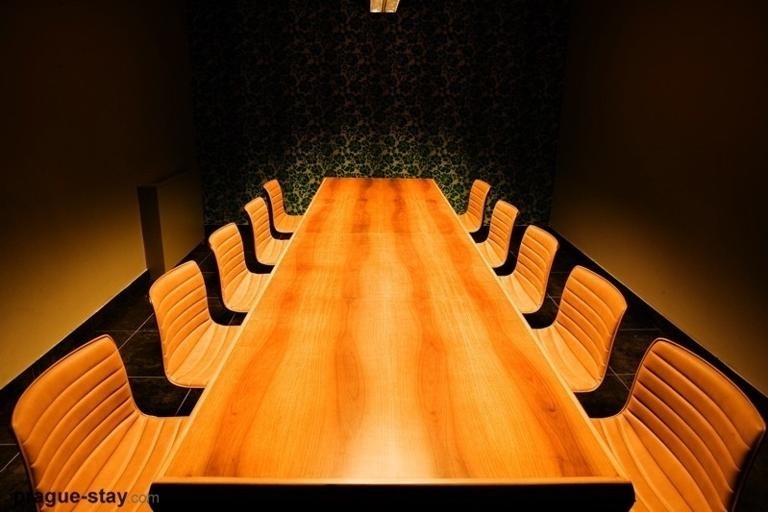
151,177,635,512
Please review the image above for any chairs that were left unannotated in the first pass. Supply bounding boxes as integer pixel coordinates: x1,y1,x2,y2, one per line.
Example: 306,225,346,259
476,200,520,269
458,179,492,233
149,261,244,388
262,179,303,233
528,265,628,393
587,337,766,512
209,223,272,312
243,197,290,266
11,333,190,512
498,225,559,314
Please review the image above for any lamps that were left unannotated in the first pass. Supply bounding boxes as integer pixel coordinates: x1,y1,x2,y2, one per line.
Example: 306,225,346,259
369,0,400,14
